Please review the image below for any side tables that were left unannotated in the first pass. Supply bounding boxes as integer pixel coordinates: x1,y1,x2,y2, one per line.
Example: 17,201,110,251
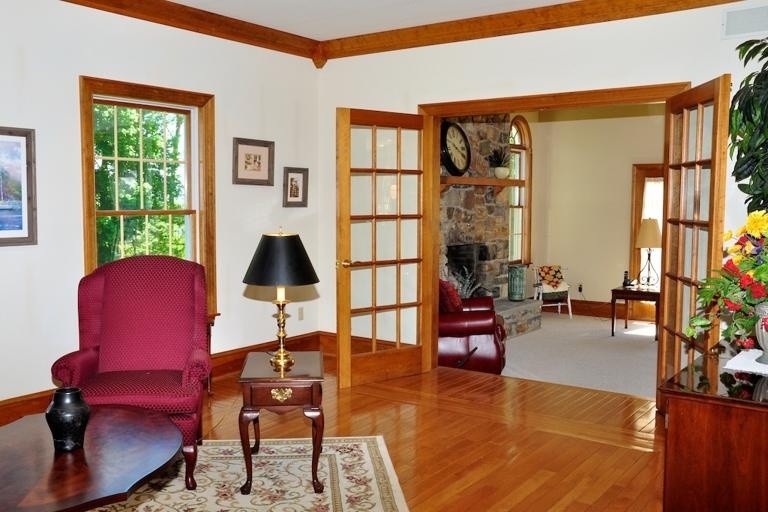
237,351,326,495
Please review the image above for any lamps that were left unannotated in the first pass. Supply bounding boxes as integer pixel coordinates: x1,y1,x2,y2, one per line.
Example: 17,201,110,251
244,228,320,372
634,217,662,291
242,284,321,320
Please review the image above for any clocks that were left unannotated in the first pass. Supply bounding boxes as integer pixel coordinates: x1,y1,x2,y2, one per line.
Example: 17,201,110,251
440,121,471,176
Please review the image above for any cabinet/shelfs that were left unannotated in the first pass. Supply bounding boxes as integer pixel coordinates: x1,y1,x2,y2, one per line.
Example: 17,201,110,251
657,334,768,512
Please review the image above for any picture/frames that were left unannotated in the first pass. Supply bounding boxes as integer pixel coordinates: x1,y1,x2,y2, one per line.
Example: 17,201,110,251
231,136,275,189
283,166,309,208
0,125,39,247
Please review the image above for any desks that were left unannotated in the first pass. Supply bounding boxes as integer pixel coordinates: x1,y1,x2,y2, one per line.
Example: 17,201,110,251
611,284,660,342
0,401,184,512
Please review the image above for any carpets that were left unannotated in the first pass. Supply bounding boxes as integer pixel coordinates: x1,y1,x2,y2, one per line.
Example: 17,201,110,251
85,434,409,512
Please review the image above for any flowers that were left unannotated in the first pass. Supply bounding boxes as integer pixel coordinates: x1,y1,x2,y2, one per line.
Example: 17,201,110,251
696,370,762,400
681,207,768,351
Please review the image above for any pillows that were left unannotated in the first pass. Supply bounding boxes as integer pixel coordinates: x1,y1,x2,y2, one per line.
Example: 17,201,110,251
438,277,463,313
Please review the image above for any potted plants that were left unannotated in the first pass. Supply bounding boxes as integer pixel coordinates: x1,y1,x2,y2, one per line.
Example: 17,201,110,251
484,146,515,180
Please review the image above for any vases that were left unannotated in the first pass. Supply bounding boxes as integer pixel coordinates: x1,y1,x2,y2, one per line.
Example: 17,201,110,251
45,386,90,453
752,301,768,365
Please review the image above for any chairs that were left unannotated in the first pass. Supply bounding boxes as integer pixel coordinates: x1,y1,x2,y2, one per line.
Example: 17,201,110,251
50,255,210,491
438,277,507,375
532,264,573,318
